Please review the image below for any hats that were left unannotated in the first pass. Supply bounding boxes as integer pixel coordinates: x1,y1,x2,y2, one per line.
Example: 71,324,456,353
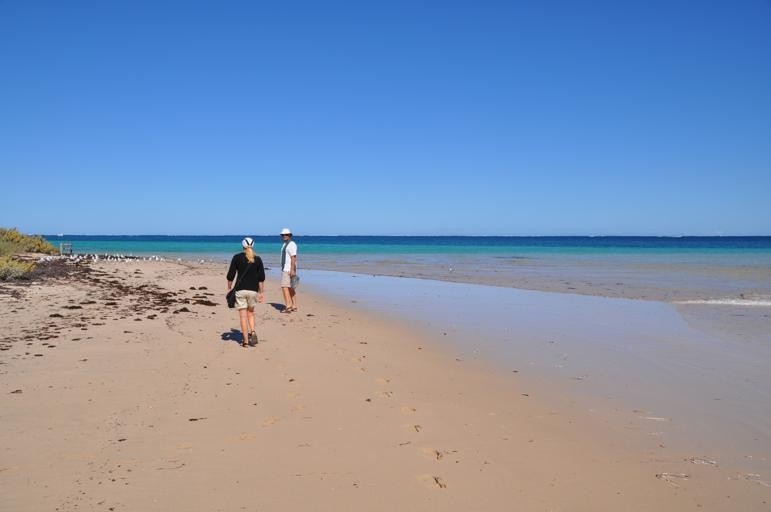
242,237,254,249
280,228,292,237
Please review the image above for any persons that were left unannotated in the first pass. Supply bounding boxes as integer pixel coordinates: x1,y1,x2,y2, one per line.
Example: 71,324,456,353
227,237,265,347
279,228,297,313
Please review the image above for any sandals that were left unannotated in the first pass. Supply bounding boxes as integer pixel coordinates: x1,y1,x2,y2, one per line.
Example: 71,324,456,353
248,331,258,346
281,307,298,313
240,343,248,348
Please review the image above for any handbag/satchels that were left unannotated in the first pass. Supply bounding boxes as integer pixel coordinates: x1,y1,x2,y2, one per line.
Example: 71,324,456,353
226,289,236,308
